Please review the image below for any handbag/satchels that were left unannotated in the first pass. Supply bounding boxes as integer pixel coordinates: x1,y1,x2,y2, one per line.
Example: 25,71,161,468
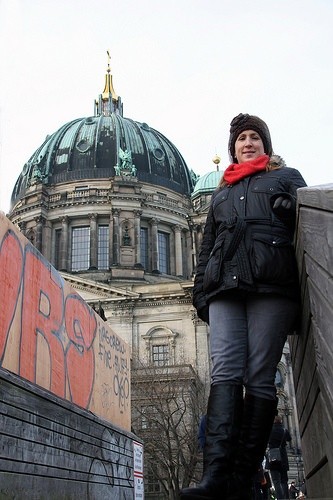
266,448,282,464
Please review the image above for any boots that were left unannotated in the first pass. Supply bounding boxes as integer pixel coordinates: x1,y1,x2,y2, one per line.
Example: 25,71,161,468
235,393,278,499
177,384,243,500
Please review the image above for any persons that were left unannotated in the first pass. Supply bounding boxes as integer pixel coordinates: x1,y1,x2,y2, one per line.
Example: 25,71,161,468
265,416,293,500
290,480,300,500
180,114,310,500
300,479,308,500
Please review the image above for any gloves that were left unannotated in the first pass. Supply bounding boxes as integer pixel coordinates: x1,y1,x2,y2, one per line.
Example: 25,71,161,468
273,196,291,209
197,306,209,324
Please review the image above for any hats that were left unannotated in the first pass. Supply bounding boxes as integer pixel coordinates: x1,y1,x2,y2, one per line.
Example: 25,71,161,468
228,113,272,162
